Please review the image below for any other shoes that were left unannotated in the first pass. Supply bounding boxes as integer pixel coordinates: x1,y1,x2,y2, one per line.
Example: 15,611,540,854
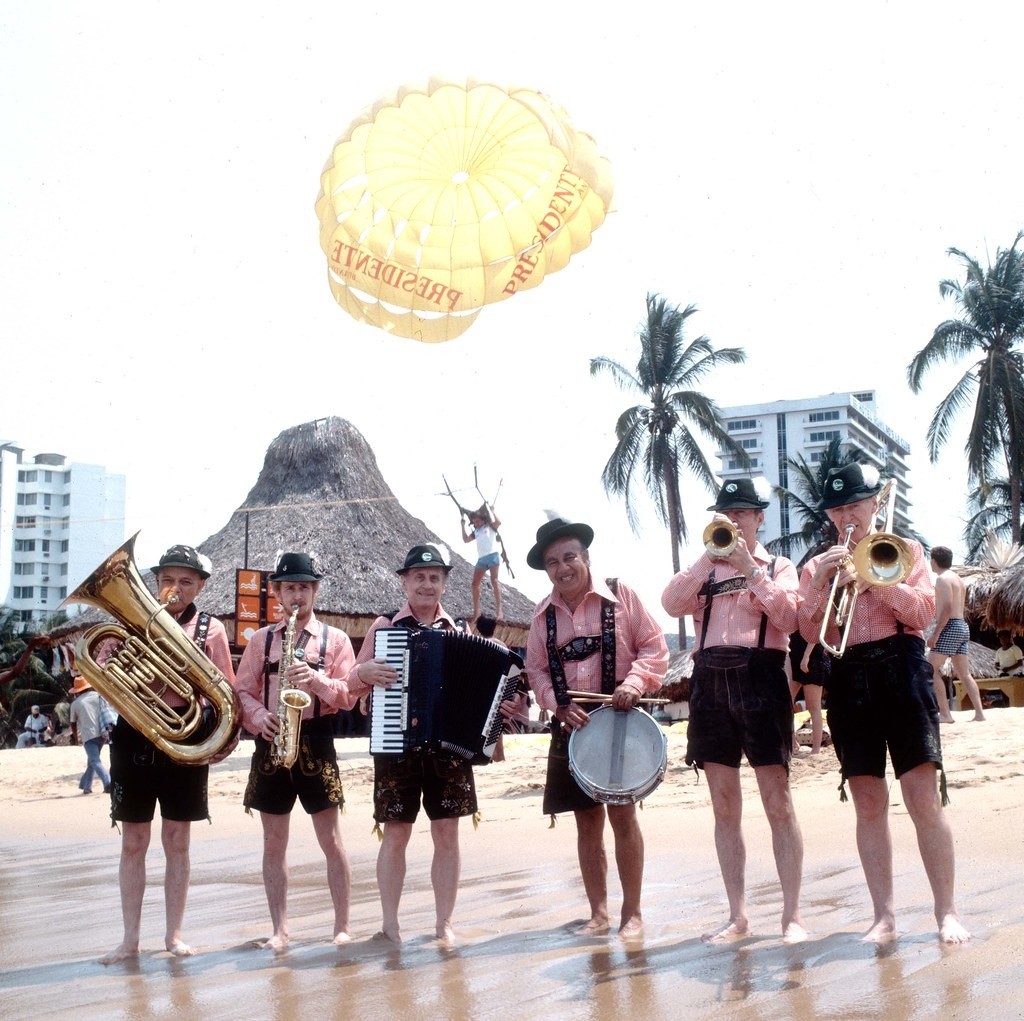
103,788,110,793
84,789,92,794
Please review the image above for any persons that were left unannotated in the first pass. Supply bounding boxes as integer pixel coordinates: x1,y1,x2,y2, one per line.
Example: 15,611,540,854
16,704,48,750
41,715,54,745
349,544,521,946
234,552,359,949
995,629,1024,678
95,546,242,962
788,630,830,754
68,675,112,793
460,506,502,623
798,464,971,945
661,478,805,946
926,547,985,723
527,519,669,938
0,636,49,716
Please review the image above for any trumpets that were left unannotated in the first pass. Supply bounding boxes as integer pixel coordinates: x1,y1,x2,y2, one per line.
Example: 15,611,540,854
701,519,740,559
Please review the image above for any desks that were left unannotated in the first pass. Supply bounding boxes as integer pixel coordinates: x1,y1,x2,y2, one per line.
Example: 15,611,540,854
952,676,1024,711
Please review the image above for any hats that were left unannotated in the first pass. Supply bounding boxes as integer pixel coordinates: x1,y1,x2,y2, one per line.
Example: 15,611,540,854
395,545,453,575
68,676,92,694
32,706,39,714
815,463,882,510
706,478,770,511
267,552,324,582
527,518,594,570
151,544,211,580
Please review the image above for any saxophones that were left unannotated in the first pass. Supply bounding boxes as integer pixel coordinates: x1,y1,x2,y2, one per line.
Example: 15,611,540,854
269,602,315,770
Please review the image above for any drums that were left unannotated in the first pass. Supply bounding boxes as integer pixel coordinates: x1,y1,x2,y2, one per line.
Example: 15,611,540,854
567,702,670,806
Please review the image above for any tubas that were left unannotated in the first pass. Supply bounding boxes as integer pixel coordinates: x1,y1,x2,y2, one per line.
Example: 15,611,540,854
56,526,247,769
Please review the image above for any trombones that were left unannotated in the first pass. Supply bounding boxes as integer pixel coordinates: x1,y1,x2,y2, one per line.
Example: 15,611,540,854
818,478,915,659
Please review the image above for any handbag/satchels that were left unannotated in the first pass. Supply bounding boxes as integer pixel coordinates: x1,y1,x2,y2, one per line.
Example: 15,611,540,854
99,695,119,740
